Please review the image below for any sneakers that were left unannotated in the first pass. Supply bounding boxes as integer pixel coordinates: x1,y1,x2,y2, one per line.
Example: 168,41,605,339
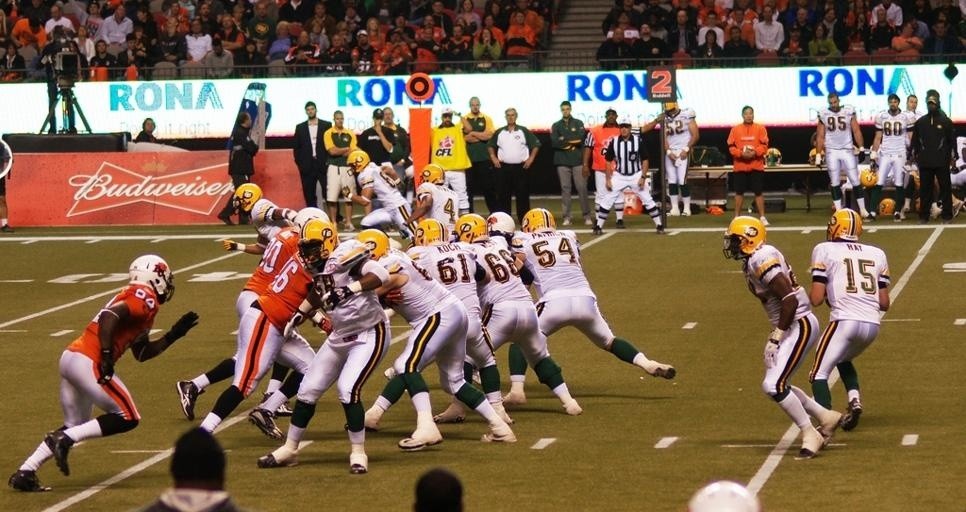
563,399,583,416
646,360,677,380
952,201,965,218
258,391,294,416
383,366,409,396
7,469,52,493
942,218,953,225
249,407,287,442
256,445,299,469
929,208,943,221
343,415,380,433
864,213,877,224
793,426,825,462
615,219,626,229
176,380,206,422
592,225,603,235
491,407,516,425
666,208,680,216
398,427,444,453
681,208,692,217
816,410,843,451
501,389,527,406
758,217,770,227
591,220,598,229
583,217,593,226
892,211,901,223
434,409,467,424
840,397,863,433
43,431,76,477
479,430,517,444
916,218,928,226
350,452,369,475
471,367,482,384
344,223,356,232
562,219,573,226
655,224,667,235
860,211,872,220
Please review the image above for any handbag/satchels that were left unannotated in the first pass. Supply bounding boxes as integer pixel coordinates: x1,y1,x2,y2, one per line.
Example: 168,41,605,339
687,146,725,168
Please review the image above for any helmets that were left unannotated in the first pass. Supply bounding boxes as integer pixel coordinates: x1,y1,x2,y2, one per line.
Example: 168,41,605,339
808,149,826,167
420,163,446,186
346,149,372,178
414,217,449,246
293,215,340,271
880,197,898,217
521,208,558,234
663,101,678,115
721,214,767,260
354,229,392,260
452,212,489,245
291,212,331,236
763,148,784,169
863,169,879,188
484,211,518,240
825,206,863,245
127,252,175,305
233,183,266,218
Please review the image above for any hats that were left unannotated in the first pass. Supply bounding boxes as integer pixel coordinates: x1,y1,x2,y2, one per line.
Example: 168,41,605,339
441,106,454,117
356,29,369,37
789,22,801,32
618,117,632,125
125,33,137,42
924,95,939,107
372,109,385,120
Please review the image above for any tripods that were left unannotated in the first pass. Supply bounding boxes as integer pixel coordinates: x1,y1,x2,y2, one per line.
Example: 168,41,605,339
39,89,93,135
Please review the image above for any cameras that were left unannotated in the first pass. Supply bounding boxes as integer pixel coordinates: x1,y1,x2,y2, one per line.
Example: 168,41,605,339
48,52,81,90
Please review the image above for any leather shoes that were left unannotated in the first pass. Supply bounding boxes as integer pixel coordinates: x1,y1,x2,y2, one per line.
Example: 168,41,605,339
217,214,234,226
239,219,253,225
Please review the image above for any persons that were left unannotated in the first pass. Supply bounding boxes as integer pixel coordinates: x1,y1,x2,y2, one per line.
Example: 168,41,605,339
501,209,676,405
204,38,233,78
431,107,472,216
816,91,876,223
25,0,48,25
487,108,541,224
406,16,448,52
457,0,482,36
408,1,431,20
446,18,474,63
138,426,238,511
483,3,508,33
99,0,126,19
266,22,296,76
324,111,357,232
447,25,468,55
126,0,151,22
365,0,394,30
199,250,334,439
224,183,296,256
582,108,664,229
278,0,312,23
664,101,698,216
408,24,448,75
433,214,582,423
476,14,505,54
506,10,536,57
342,151,418,241
401,165,460,242
428,1,453,40
292,101,346,223
337,5,363,37
352,29,377,75
131,117,158,145
55,2,80,32
185,17,212,61
101,6,132,55
359,110,396,218
463,97,496,215
904,94,924,142
404,153,414,208
192,4,218,39
0,44,26,84
344,220,515,434
473,29,500,73
337,22,357,51
382,107,410,196
175,208,329,421
0,0,18,23
35,23,79,134
470,210,532,383
869,93,916,222
379,31,412,74
0,140,15,234
308,19,330,54
808,207,890,431
195,0,224,17
257,218,391,473
218,114,258,224
230,6,247,37
367,18,385,75
87,41,119,81
550,101,593,226
238,40,267,78
10,16,46,76
178,0,196,23
386,13,415,43
70,0,103,40
161,0,178,12
283,32,322,77
0,9,10,76
727,106,772,228
288,22,305,46
249,3,276,55
722,215,843,460
308,3,334,39
320,34,352,77
6,255,201,493
30,53,46,82
70,40,89,81
591,118,665,236
157,3,189,34
900,137,966,219
527,0,552,44
158,17,187,66
356,230,517,451
214,14,245,67
510,0,548,57
223,0,254,18
595,1,966,69
905,97,958,225
132,26,160,62
45,6,76,42
118,35,152,83
132,10,159,40
73,26,95,67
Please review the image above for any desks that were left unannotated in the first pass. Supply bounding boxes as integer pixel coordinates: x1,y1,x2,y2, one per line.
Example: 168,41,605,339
686,162,827,213
